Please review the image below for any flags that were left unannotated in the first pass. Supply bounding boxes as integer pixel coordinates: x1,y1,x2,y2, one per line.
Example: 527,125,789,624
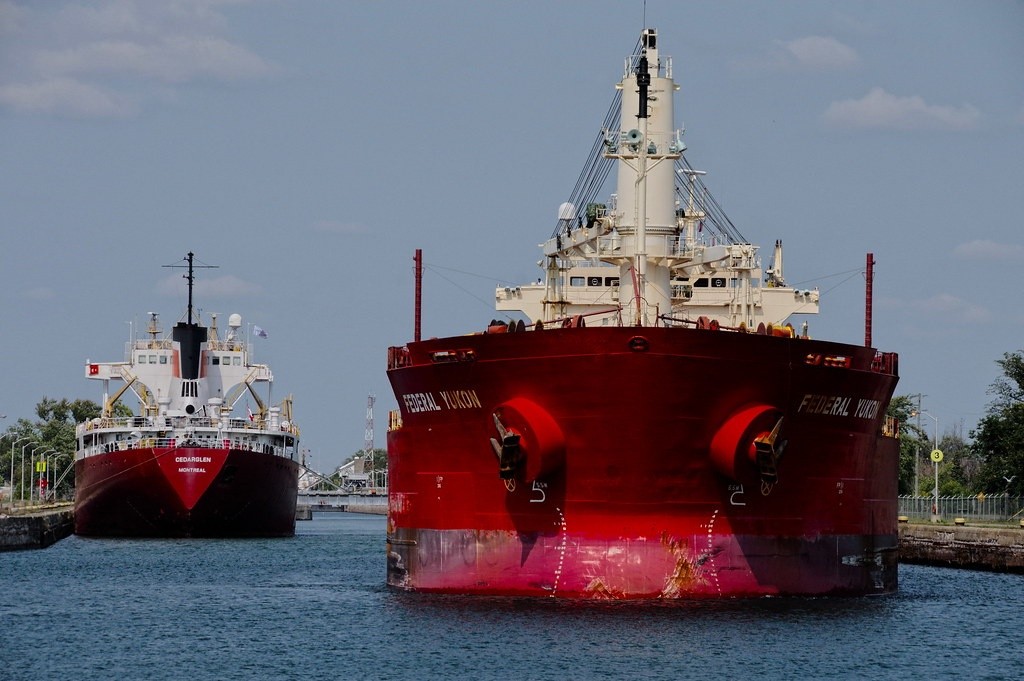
247,404,253,422
253,325,269,338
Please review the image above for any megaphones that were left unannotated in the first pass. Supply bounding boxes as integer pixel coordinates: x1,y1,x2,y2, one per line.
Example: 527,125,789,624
627,129,642,143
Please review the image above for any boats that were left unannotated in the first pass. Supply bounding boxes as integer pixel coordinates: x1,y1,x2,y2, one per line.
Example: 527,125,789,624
386,28,900,599
75,252,299,537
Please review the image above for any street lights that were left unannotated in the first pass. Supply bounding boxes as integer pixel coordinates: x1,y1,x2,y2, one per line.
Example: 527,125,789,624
47,453,61,489
54,454,66,498
912,411,938,515
22,442,39,500
10,437,30,502
39,450,56,493
30,446,47,500
